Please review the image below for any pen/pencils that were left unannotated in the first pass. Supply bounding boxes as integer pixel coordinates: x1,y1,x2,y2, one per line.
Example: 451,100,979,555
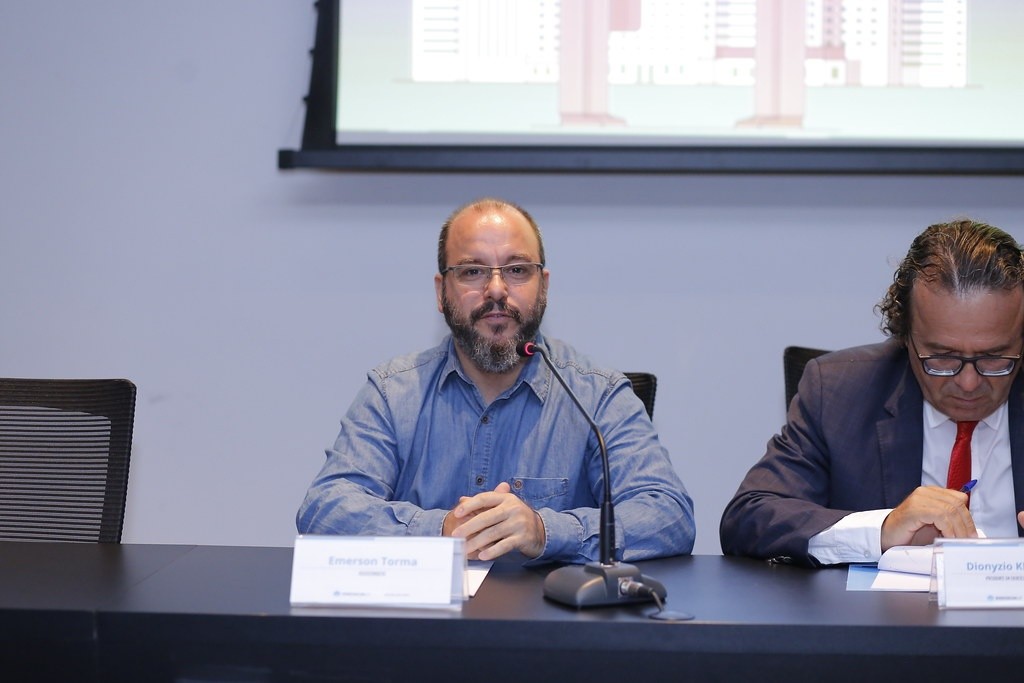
959,479,978,494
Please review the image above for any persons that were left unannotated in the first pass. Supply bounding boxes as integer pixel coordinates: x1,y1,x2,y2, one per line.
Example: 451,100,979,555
295,196,698,568
719,219,1024,571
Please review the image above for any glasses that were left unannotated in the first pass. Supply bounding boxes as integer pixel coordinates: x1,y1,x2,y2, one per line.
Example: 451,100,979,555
442,262,543,287
909,333,1022,376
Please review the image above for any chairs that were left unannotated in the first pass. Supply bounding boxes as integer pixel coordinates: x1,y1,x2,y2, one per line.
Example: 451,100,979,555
0,377,139,544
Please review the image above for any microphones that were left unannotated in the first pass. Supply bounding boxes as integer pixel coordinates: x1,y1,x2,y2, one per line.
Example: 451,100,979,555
516,340,666,607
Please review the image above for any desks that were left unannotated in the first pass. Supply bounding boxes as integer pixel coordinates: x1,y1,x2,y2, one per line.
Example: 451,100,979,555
0,545,1024,683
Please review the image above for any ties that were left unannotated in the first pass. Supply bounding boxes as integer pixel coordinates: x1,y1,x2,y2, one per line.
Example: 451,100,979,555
945,421,979,511
